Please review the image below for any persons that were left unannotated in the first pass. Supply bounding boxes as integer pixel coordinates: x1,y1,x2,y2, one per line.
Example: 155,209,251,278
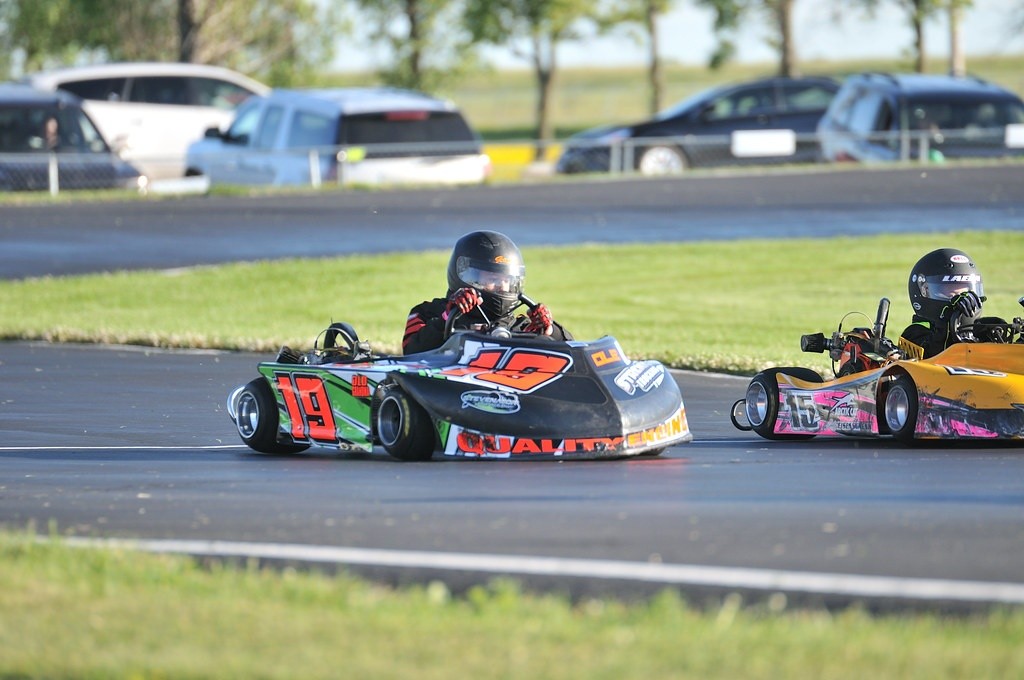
402,230,573,356
900,248,1024,362
30,118,75,148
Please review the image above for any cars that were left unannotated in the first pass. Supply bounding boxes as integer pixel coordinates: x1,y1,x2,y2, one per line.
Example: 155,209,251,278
1,75,149,196
555,66,842,178
29,58,276,179
182,83,486,195
814,72,1023,163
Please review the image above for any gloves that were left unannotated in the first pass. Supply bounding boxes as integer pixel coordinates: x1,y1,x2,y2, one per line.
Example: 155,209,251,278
950,290,987,317
527,304,553,329
446,287,484,317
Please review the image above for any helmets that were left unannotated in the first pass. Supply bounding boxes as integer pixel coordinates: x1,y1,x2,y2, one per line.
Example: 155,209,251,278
448,230,525,319
908,248,982,323
